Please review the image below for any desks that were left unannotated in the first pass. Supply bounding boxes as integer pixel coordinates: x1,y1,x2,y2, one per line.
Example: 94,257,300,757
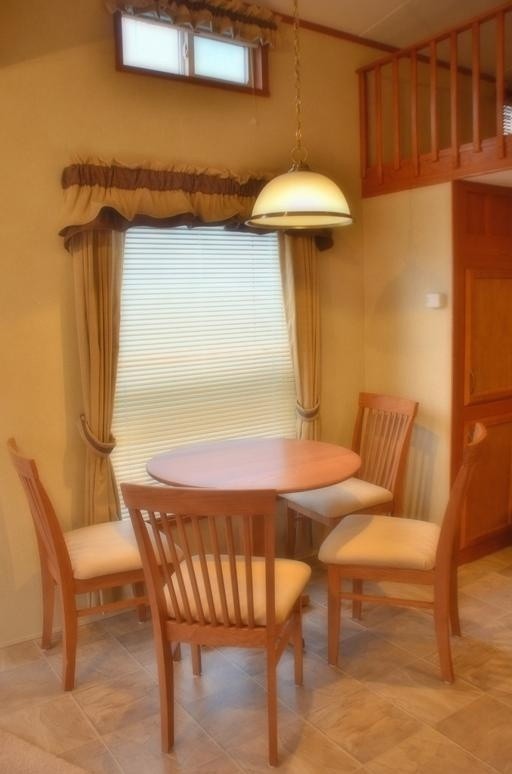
146,438,360,608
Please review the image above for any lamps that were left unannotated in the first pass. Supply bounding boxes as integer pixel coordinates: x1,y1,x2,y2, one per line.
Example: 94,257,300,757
244,2,354,231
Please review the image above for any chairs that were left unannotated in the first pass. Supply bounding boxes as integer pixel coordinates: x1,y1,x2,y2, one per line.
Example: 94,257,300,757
7,435,183,690
279,391,420,622
318,422,488,685
118,482,314,766
